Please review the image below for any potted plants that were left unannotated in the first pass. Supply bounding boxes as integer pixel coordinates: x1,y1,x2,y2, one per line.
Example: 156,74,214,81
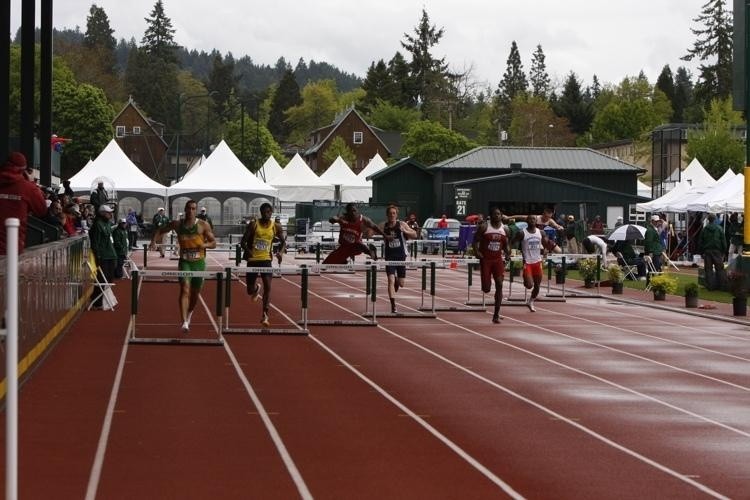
510,257,749,317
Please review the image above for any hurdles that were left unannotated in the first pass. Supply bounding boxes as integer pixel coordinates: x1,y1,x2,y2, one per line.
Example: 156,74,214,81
229,234,280,277
381,240,446,271
546,254,603,298
296,264,380,326
463,256,530,306
142,238,448,284
129,270,227,344
221,266,312,334
294,234,375,273
170,233,180,260
360,259,438,318
506,256,567,302
417,256,487,312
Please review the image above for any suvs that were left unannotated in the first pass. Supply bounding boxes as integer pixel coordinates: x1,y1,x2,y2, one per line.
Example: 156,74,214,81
421,218,464,254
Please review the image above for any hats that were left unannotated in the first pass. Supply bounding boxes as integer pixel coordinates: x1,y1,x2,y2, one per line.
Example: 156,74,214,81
201,207,206,210
158,207,165,211
651,215,660,222
99,205,114,212
7,152,27,166
568,215,575,221
119,218,127,224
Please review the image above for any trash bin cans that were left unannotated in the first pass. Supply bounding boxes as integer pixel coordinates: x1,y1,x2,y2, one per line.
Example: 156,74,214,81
294,218,307,249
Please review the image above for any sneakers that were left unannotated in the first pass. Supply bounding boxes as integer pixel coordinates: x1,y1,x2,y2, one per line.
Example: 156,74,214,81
251,283,261,302
261,317,271,326
394,277,399,292
391,305,397,314
370,243,378,261
182,319,191,331
526,302,536,312
492,314,501,324
86,301,103,310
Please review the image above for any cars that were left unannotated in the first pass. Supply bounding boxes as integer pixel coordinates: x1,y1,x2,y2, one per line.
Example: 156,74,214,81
508,221,528,229
310,221,342,251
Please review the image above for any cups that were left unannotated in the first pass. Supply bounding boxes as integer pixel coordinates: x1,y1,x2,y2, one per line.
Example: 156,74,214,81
81,220,87,228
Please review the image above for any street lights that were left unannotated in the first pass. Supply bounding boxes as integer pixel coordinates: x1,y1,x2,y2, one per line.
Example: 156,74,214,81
544,125,554,147
